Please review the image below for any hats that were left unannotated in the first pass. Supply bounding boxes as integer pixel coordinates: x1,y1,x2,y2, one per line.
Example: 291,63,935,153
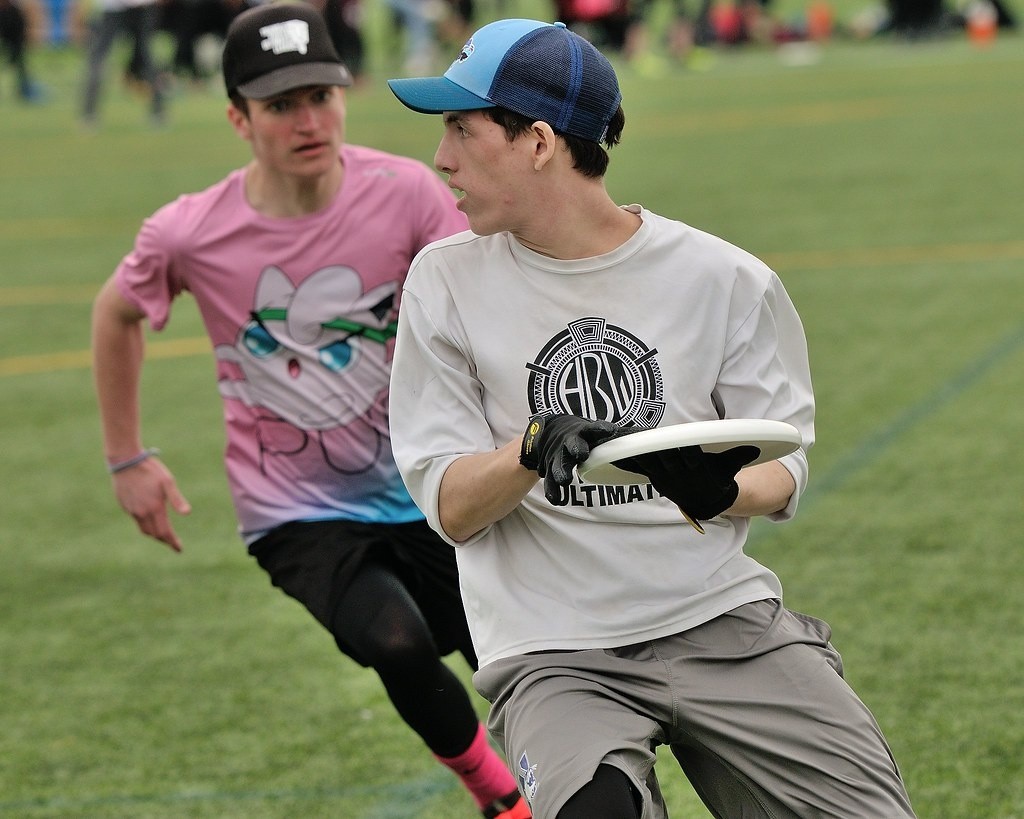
223,3,354,101
387,18,622,143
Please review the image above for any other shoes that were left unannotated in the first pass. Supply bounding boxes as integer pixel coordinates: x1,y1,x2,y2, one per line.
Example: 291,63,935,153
478,780,534,819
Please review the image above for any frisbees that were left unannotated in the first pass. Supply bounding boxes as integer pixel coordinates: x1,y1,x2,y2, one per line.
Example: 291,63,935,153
576,416,804,485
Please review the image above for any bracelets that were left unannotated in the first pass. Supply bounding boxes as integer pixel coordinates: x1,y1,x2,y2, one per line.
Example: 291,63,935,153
112,447,161,476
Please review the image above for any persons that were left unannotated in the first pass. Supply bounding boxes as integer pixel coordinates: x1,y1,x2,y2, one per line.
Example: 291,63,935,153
1,0,1023,119
384,17,917,819
92,0,531,819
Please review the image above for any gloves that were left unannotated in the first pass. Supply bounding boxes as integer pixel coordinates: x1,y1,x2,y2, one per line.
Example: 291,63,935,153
610,443,761,535
519,413,656,506
